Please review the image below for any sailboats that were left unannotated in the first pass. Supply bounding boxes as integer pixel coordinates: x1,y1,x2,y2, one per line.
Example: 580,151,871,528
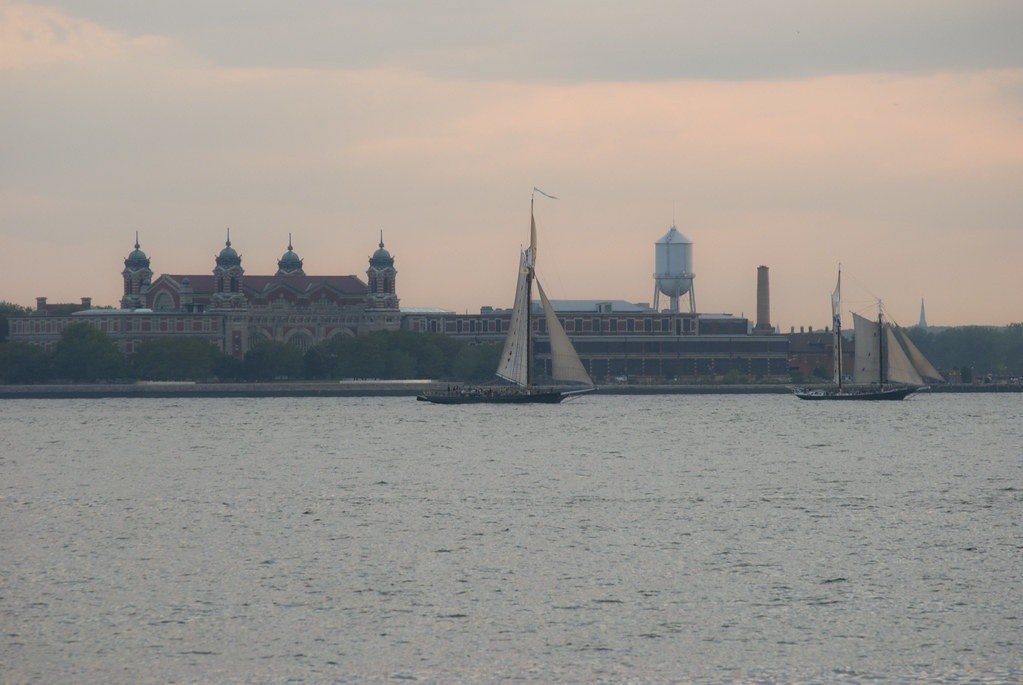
791,260,944,402
416,187,598,406
